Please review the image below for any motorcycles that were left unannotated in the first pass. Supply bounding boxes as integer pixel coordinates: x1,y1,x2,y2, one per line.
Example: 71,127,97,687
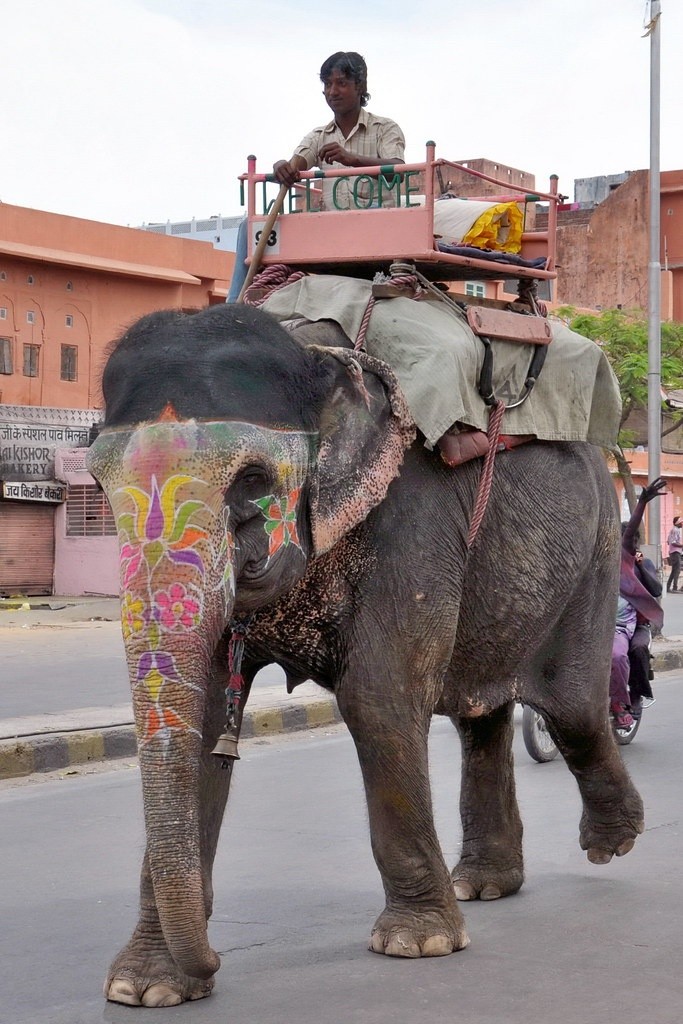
522,630,656,763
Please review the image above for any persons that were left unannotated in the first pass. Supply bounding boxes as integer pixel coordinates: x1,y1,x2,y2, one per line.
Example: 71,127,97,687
610,475,669,728
667,515,683,594
271,51,406,210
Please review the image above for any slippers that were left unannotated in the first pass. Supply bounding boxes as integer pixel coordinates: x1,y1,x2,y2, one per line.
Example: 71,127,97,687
613,710,633,728
629,703,643,719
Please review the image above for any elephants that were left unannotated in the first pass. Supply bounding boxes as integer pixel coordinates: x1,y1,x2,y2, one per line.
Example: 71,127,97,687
85,275,643,1009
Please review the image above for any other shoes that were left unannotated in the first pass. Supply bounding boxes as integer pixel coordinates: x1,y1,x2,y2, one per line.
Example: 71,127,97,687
667,588,671,592
671,590,681,594
